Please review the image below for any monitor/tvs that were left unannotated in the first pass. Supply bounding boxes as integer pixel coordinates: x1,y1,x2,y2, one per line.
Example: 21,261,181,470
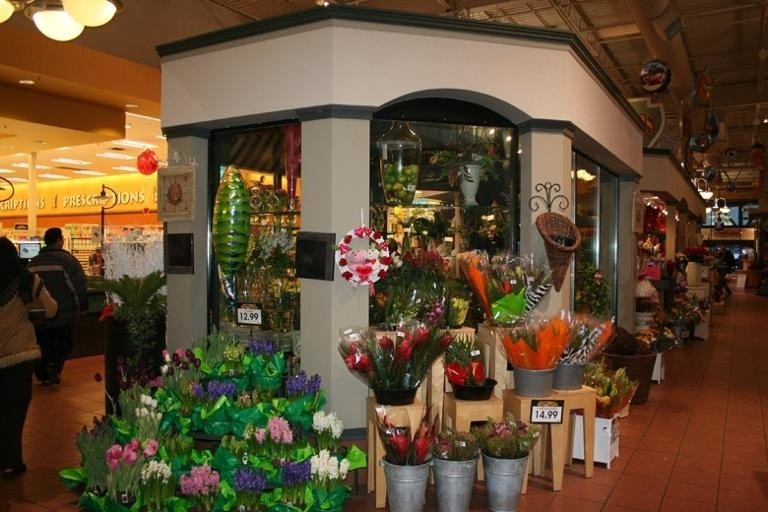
19,243,40,262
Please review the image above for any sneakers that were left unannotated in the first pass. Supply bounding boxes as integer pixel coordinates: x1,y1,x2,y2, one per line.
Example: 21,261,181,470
4,462,28,478
38,359,60,386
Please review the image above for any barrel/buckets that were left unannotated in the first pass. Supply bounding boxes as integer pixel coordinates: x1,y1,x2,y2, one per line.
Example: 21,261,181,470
432,453,480,512
601,351,657,406
480,449,530,512
379,455,433,512
687,261,701,287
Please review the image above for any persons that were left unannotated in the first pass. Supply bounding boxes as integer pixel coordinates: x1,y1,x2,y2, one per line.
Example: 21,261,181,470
716,245,735,296
25,227,89,386
0,237,59,480
90,249,105,276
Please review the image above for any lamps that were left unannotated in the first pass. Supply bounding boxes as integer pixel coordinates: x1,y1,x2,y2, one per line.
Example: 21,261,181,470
0,0,128,43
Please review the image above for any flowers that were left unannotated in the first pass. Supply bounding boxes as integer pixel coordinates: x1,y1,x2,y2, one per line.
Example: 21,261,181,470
341,327,453,390
634,293,711,350
503,318,599,359
76,338,349,501
378,420,535,464
383,251,553,327
349,249,381,281
582,364,634,417
640,239,712,292
